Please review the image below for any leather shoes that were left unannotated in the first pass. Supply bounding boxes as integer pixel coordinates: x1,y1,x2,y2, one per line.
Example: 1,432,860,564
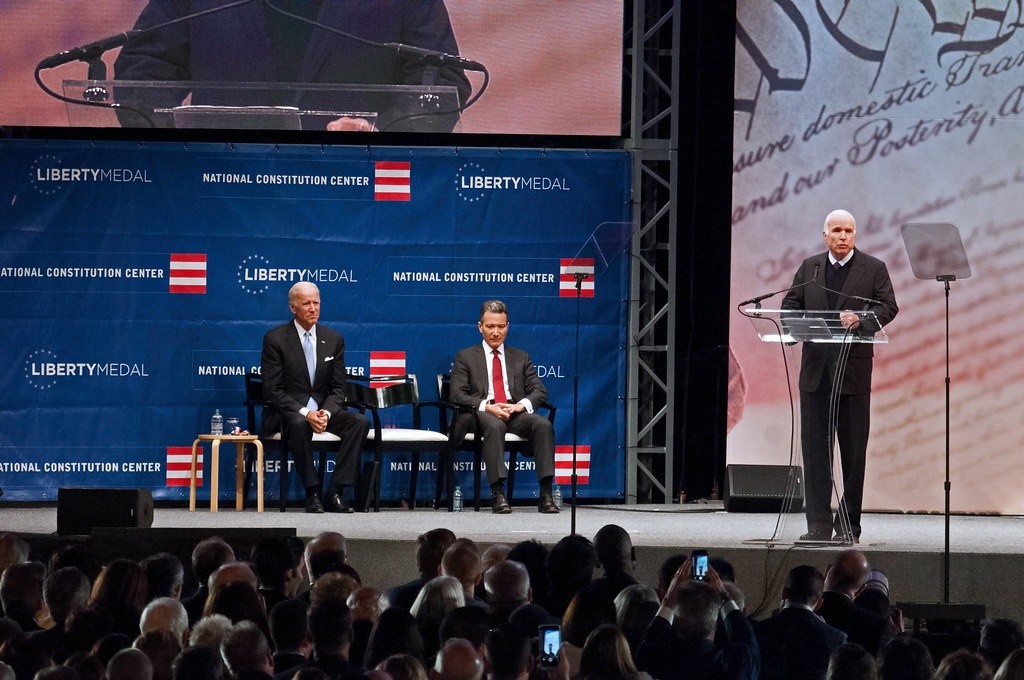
322,493,355,513
538,491,559,513
833,533,859,543
305,494,322,513
799,528,831,540
491,489,512,513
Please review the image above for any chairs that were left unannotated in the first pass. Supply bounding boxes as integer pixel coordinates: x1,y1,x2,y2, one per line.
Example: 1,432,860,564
242,372,559,512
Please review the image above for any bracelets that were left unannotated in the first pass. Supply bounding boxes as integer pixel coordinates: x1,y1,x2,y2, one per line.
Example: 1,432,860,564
661,597,676,609
718,590,733,607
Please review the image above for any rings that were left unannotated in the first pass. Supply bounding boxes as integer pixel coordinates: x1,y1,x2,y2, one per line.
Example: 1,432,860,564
324,428,325,430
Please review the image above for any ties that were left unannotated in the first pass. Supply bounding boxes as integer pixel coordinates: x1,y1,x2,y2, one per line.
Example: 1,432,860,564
302,331,319,411
834,262,841,269
492,350,508,404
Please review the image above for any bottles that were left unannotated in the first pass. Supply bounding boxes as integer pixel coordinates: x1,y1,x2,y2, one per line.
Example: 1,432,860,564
553,486,562,509
210,409,223,436
226,417,240,435
452,486,463,512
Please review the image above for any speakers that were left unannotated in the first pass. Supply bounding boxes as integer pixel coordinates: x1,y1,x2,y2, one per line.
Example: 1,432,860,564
56,488,155,537
721,464,805,512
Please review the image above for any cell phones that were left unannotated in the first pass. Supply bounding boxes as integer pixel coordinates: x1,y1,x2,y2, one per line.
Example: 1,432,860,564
691,550,709,581
538,623,562,666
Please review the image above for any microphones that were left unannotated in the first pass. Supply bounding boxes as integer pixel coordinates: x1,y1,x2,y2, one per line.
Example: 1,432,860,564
739,261,884,306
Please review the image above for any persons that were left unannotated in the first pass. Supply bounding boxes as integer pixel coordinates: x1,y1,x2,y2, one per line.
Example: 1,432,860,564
261,281,371,513
113,0,473,131
0,524,1024,680
780,209,899,546
450,300,559,513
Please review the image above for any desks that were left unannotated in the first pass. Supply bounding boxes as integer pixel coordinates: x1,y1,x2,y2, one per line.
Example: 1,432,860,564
189,433,264,512
